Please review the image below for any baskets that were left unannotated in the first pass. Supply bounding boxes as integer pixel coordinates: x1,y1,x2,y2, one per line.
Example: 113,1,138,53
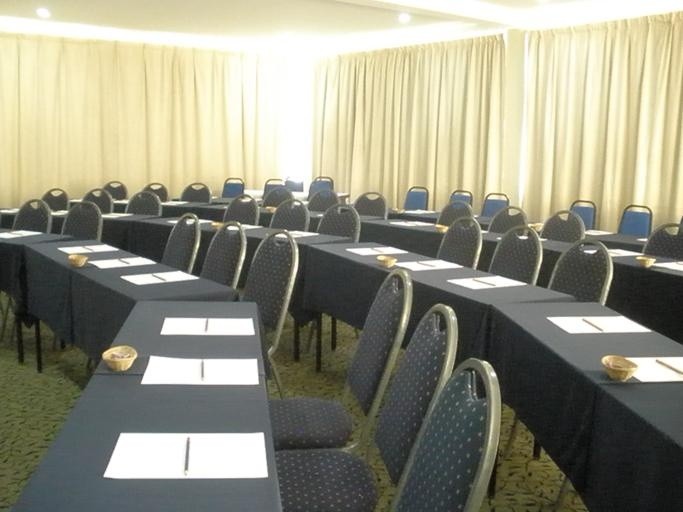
527,222,543,232
102,344,139,371
633,256,656,268
266,207,277,214
434,224,448,234
600,354,639,381
391,208,404,214
68,254,89,267
211,222,223,230
376,255,397,268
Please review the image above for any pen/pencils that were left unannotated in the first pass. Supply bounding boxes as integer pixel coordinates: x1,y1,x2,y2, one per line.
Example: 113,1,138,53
371,248,383,253
472,278,496,286
417,260,436,267
83,246,92,250
582,318,603,331
201,360,204,377
205,319,208,331
656,359,683,375
152,274,166,281
118,259,129,264
185,438,190,476
